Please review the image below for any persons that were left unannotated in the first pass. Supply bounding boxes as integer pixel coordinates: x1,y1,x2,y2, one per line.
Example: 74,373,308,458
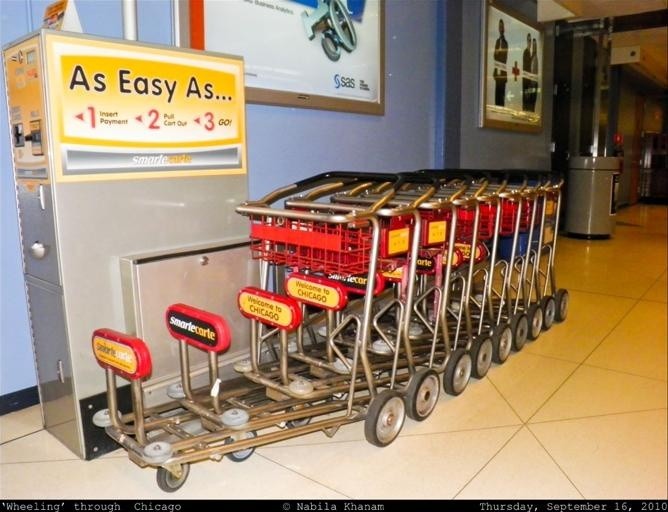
494,20,508,105
523,34,532,110
529,39,538,111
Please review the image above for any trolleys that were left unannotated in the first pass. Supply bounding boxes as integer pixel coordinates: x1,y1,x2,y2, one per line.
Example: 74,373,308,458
92,169,567,493
